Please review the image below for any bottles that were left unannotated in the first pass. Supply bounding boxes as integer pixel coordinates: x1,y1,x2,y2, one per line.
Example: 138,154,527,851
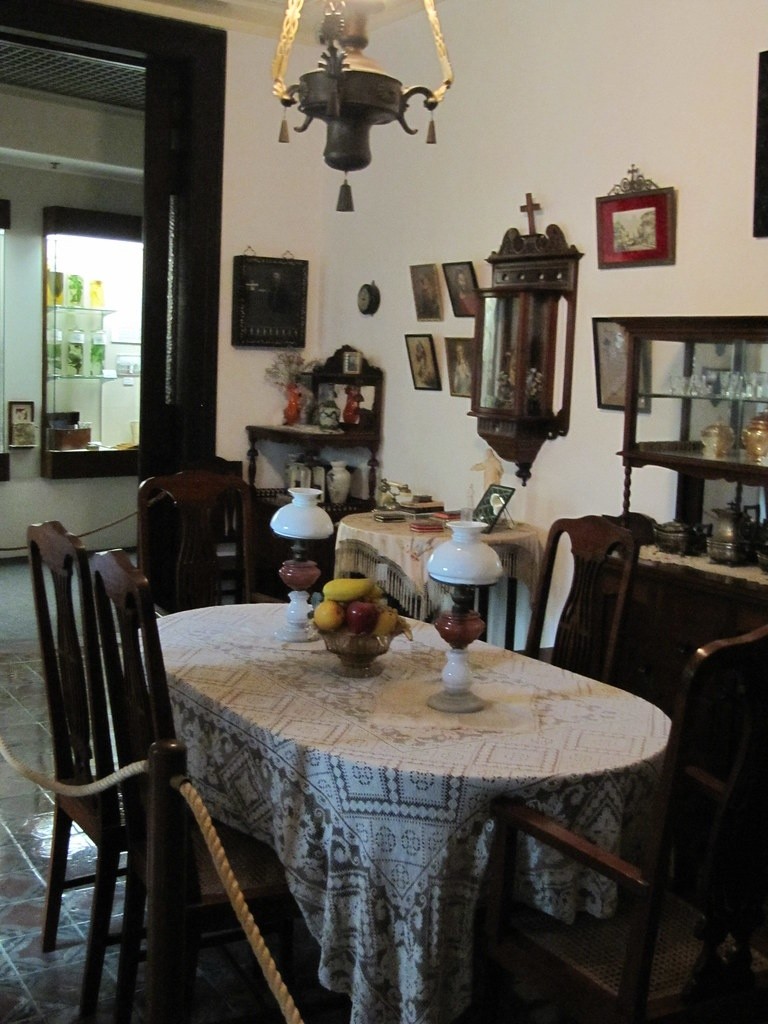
326,460,351,505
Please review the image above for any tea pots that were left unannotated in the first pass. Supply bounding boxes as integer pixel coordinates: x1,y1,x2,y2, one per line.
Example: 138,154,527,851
712,501,761,544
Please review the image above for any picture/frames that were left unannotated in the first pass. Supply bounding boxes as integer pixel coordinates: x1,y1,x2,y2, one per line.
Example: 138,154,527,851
343,351,362,375
404,334,442,391
592,317,652,414
596,163,676,269
468,484,516,534
444,336,474,397
409,263,443,322
441,261,480,317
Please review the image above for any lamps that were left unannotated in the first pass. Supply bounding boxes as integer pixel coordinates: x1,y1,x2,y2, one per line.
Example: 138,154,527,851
268,487,333,642
273,0,453,212
424,520,505,712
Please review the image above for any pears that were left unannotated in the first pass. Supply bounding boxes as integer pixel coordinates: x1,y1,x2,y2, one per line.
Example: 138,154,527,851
314,600,344,632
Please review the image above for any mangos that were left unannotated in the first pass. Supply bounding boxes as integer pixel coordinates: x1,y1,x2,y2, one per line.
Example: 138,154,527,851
323,578,376,602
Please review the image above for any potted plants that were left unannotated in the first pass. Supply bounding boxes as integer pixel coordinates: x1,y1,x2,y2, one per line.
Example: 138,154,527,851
317,401,341,429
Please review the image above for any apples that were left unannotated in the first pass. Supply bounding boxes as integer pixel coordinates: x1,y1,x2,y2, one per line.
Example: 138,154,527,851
346,600,377,636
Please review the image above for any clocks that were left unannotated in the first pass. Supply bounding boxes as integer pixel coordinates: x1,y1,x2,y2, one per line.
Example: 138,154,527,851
356,284,381,315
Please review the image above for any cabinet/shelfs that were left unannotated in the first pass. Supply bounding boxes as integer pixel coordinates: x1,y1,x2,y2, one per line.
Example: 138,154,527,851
610,316,768,900
39,205,142,479
245,343,384,604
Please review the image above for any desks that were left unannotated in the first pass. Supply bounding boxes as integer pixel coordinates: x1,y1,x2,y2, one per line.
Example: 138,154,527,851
117,604,672,1024
333,510,545,651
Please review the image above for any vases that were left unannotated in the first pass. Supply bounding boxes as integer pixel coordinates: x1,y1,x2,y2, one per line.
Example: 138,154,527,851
343,383,365,425
282,384,303,425
326,460,352,507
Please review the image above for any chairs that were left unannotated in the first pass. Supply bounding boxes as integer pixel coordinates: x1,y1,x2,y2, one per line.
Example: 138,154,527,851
87,548,307,1024
524,516,638,685
25,518,263,1024
470,622,768,1024
136,470,251,618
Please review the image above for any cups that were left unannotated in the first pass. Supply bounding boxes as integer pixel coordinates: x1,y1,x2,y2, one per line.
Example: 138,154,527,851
47,271,64,306
668,370,768,400
88,280,106,307
67,274,84,306
89,329,108,376
43,328,63,376
66,330,84,375
